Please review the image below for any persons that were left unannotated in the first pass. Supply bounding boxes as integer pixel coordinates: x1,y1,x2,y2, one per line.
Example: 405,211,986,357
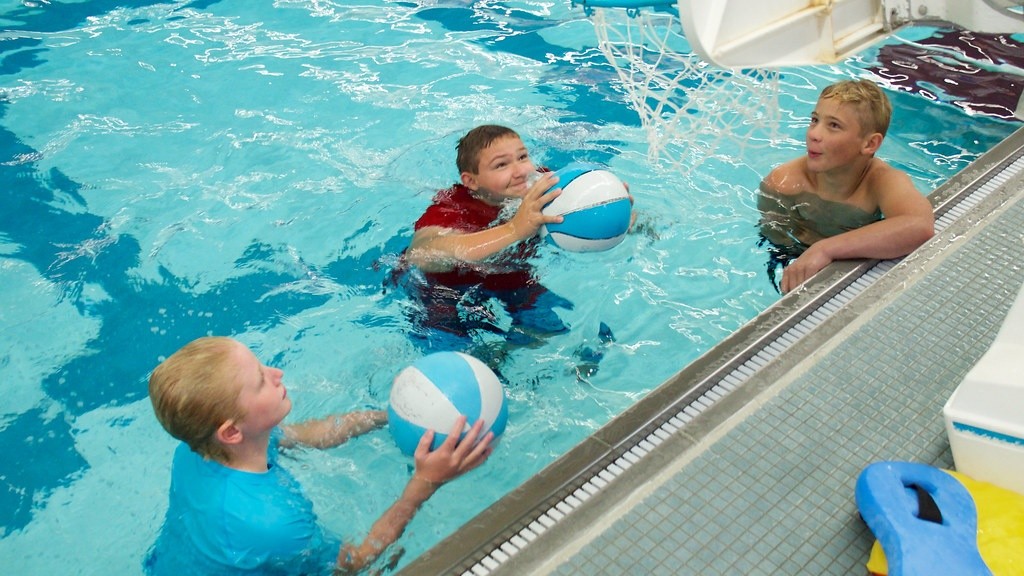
141,331,495,574
403,123,638,387
753,77,936,295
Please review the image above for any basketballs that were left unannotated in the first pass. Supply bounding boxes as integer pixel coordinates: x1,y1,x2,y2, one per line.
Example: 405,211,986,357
537,163,632,254
388,351,509,468
